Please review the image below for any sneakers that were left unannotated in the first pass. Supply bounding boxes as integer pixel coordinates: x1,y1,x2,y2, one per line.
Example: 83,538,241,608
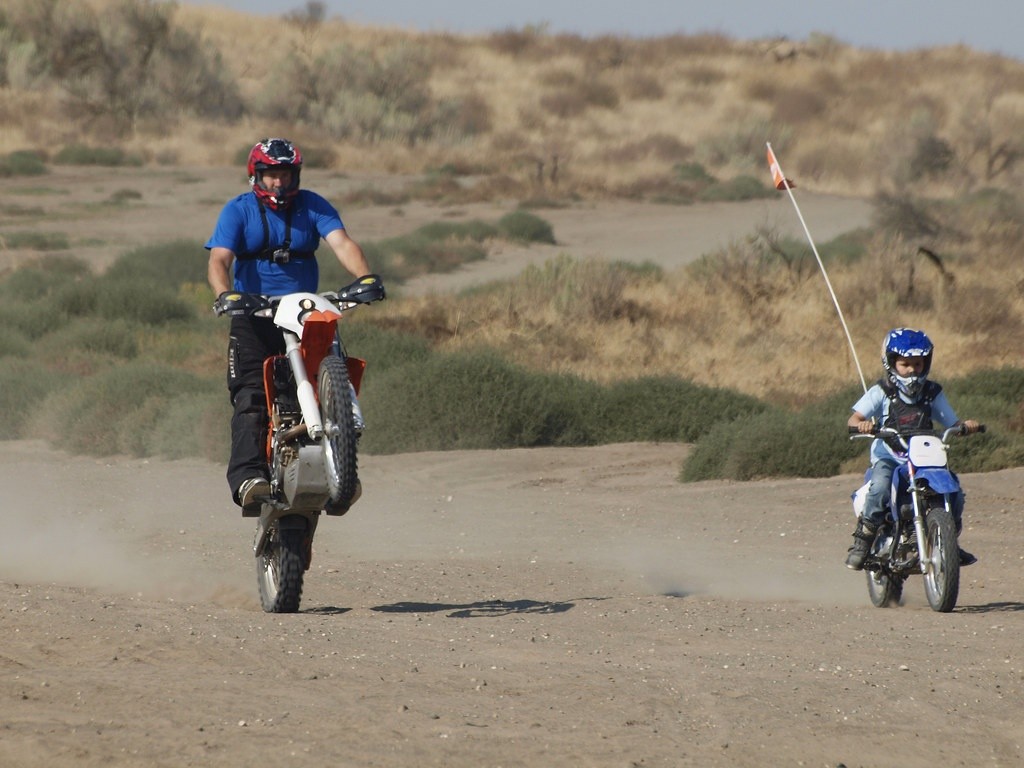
326,479,363,517
236,477,270,517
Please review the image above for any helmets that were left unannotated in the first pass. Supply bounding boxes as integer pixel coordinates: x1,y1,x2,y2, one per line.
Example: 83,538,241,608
247,138,301,211
881,327,933,399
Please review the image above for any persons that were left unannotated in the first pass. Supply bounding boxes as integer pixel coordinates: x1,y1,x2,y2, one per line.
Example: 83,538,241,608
843,326,978,571
204,139,385,519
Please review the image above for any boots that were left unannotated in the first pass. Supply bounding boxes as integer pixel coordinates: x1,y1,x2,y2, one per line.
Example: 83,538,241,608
845,515,879,571
954,518,976,566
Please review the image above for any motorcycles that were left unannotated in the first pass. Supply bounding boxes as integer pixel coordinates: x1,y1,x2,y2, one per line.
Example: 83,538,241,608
211,274,389,615
847,412,987,613
